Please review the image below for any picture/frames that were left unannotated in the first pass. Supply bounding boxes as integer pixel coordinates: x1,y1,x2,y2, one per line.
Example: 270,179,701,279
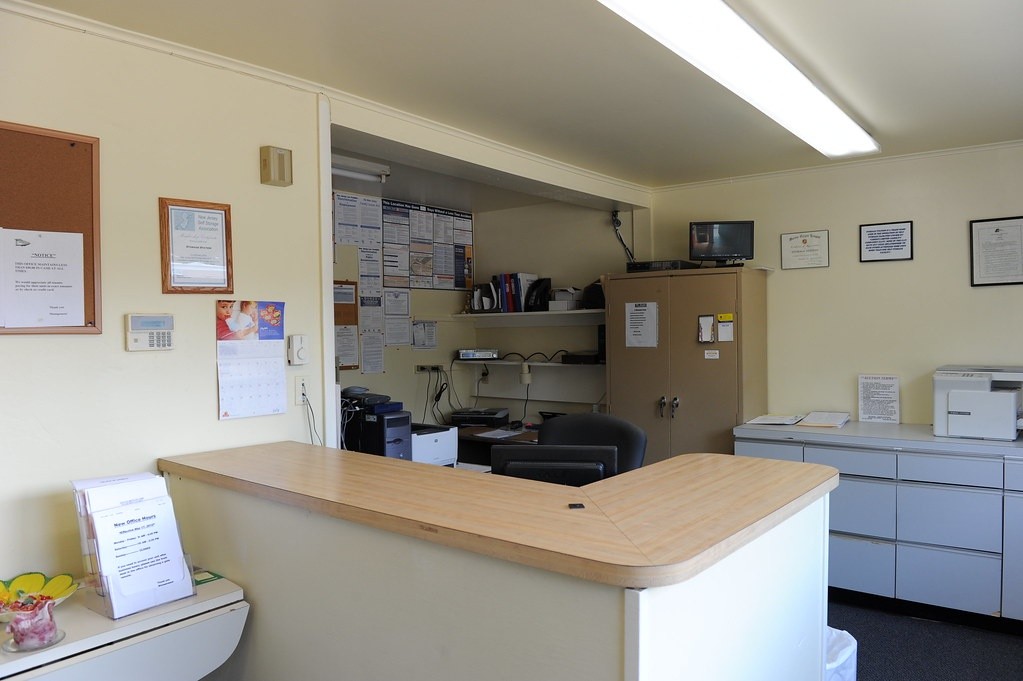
160,197,233,295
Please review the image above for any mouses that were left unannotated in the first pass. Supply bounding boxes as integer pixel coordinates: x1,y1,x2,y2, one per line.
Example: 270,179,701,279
510,421,523,429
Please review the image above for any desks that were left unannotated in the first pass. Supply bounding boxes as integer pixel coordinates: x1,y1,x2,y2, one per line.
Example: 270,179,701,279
0,560,250,681
447,420,543,444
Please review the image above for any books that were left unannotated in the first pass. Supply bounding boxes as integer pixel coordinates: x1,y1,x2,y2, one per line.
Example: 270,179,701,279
499,272,538,312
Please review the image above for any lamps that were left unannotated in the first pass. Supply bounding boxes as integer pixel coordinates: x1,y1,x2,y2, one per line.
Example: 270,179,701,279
332,152,391,183
519,361,533,385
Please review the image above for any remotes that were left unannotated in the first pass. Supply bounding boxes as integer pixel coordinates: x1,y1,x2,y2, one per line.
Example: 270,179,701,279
716,263,744,268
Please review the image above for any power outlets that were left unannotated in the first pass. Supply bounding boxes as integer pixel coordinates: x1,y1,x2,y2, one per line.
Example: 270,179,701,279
482,368,489,384
296,375,313,404
415,363,444,374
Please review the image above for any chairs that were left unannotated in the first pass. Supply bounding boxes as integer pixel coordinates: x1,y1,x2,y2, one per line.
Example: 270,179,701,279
536,415,647,474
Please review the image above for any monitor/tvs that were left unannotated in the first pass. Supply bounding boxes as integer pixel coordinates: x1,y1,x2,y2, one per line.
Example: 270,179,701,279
491,445,617,487
690,221,754,266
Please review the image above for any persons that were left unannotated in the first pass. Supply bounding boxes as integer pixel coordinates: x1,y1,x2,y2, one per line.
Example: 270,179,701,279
217,300,258,340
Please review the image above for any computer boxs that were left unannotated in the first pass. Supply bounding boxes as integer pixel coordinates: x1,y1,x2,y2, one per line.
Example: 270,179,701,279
340,410,412,461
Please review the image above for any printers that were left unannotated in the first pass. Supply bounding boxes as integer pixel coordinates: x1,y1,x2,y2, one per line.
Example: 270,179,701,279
411,423,458,469
451,407,510,428
933,365,1023,442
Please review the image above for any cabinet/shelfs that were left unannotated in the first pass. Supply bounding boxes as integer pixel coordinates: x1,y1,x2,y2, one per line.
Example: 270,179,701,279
607,265,770,463
732,419,1023,624
459,310,609,404
